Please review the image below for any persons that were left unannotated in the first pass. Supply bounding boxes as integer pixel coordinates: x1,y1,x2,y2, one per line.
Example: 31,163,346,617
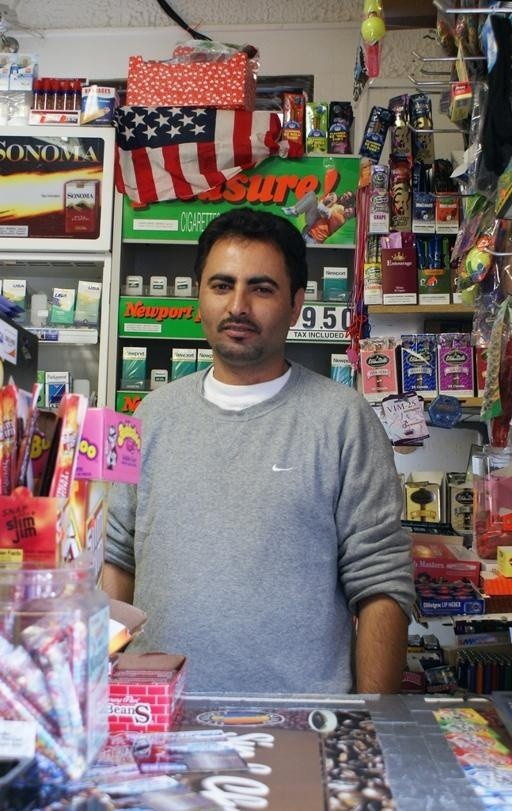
303,201,357,245
96,205,424,699
280,188,356,228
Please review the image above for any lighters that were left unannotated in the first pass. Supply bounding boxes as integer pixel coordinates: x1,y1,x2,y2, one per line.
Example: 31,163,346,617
451,646,512,698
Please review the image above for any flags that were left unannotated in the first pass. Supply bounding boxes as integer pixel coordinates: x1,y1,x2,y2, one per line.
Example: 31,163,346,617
112,104,283,205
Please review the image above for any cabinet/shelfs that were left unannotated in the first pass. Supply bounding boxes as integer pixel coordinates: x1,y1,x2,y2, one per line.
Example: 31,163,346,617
3,126,109,409
116,144,357,421
361,304,483,678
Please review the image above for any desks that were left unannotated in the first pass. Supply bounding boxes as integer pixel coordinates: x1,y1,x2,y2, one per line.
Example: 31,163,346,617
84,684,509,809
